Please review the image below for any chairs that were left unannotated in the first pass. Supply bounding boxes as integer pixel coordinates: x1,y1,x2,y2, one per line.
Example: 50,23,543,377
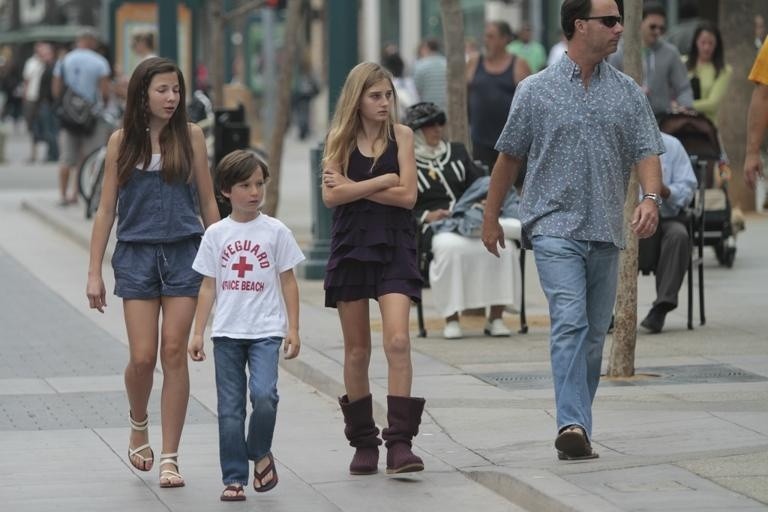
402,159,528,338
604,154,706,331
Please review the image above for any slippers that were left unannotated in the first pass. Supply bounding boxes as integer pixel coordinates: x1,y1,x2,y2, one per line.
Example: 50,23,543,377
252,452,278,493
220,485,246,501
555,426,593,456
557,451,599,461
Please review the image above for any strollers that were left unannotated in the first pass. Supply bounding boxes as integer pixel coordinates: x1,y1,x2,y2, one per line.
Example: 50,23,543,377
647,93,746,269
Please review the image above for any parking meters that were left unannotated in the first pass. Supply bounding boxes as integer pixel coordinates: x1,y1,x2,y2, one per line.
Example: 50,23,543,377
210,104,251,219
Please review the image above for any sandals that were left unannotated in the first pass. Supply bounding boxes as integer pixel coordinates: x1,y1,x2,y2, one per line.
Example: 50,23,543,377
159,452,185,488
127,410,154,471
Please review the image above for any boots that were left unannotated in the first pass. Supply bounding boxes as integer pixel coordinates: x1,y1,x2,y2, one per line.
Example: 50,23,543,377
337,393,382,474
381,394,426,474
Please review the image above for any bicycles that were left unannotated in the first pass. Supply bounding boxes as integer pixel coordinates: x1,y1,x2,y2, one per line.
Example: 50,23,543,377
76,84,217,222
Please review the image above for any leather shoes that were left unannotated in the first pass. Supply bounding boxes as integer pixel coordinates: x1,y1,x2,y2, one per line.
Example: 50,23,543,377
483,315,513,338
640,306,667,334
443,319,464,340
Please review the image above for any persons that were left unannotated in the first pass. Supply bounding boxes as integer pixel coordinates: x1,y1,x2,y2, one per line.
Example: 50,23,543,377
383,21,571,194
610,6,695,122
480,0,664,462
1,32,162,206
403,101,522,338
185,148,305,502
677,23,733,164
317,57,431,479
83,56,229,492
744,34,768,191
609,132,699,333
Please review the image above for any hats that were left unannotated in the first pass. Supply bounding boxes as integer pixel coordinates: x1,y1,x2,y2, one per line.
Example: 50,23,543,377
400,103,447,132
424,117,445,128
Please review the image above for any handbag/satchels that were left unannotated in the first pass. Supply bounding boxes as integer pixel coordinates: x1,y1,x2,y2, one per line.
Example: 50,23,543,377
55,61,97,135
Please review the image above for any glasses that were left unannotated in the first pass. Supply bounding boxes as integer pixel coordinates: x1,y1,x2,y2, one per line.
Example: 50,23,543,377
650,24,665,33
579,15,623,28
